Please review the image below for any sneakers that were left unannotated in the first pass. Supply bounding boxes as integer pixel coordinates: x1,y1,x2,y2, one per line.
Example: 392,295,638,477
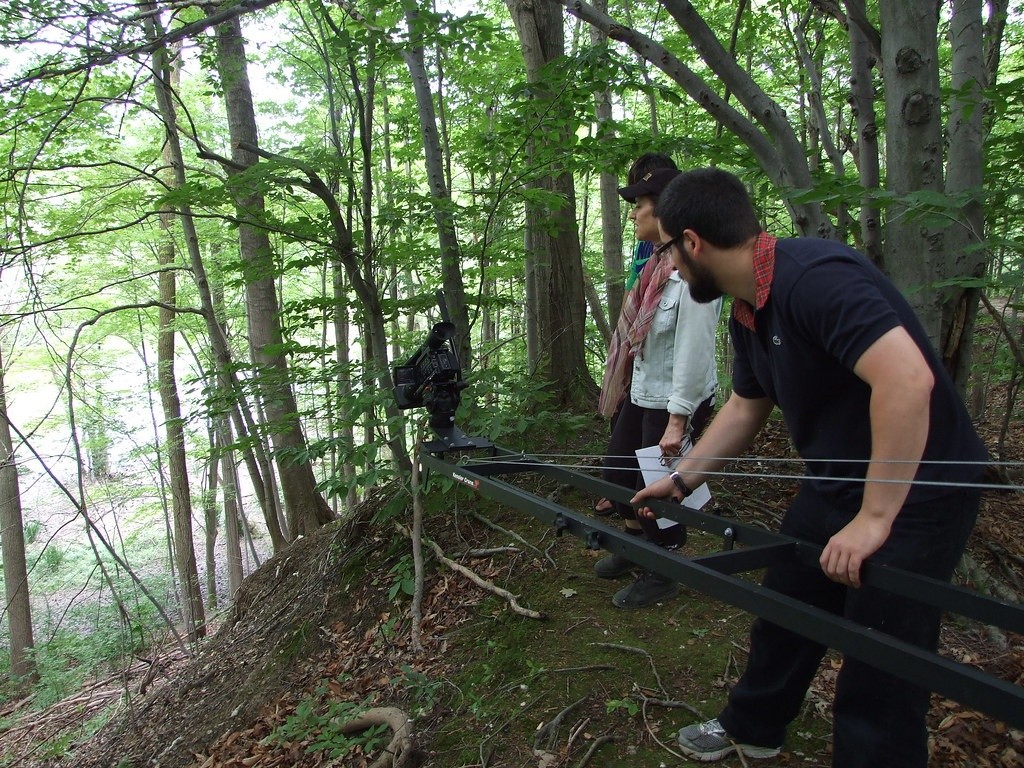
677,718,781,760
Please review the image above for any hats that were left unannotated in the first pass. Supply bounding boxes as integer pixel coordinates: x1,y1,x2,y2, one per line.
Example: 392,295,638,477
617,168,684,201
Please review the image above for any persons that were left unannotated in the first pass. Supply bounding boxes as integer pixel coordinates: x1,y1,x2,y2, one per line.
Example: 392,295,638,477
595,153,678,516
594,168,725,608
630,168,988,768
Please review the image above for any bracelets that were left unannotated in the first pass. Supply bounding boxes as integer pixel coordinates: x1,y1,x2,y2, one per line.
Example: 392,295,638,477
669,469,693,497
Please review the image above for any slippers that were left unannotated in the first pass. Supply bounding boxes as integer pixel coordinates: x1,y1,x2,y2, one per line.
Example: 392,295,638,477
596,497,616,516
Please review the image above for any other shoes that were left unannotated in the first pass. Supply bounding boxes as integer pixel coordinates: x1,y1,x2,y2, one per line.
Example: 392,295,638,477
612,569,677,608
593,553,634,577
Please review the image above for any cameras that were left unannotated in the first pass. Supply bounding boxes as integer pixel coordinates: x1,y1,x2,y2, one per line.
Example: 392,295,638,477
391,322,469,409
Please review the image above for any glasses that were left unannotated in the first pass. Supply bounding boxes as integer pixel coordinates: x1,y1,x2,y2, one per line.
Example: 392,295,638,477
653,238,683,263
